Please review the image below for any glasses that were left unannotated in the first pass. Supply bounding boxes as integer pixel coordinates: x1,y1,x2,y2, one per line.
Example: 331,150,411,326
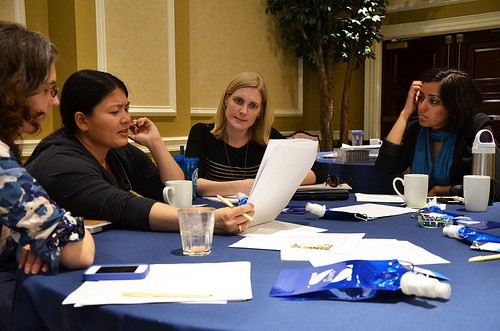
44,82,59,98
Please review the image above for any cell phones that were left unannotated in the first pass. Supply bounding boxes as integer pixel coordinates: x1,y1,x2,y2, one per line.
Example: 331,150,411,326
82,264,150,282
437,198,463,205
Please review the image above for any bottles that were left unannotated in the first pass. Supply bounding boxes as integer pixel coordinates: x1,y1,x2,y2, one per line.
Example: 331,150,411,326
472,129,496,206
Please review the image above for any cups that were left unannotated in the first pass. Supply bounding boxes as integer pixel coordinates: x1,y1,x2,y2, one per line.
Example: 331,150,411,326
352,130,362,146
392,174,429,208
163,180,192,207
370,138,382,145
174,155,200,201
463,175,490,212
177,207,215,256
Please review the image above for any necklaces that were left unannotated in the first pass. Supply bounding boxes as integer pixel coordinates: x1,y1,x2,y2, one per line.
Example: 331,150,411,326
221,132,248,181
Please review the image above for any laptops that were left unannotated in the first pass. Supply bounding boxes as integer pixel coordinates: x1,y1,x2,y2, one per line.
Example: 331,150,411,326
292,189,350,201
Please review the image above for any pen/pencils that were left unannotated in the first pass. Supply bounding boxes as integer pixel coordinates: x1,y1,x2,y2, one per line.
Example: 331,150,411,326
216,194,258,225
468,254,500,263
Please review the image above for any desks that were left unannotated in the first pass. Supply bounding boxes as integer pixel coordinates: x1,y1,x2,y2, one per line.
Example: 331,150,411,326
27,198,500,331
315,144,379,192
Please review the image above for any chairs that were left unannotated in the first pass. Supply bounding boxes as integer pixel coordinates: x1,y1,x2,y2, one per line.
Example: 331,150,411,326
284,130,321,151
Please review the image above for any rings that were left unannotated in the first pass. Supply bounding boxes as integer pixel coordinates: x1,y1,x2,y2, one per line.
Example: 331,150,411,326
238,224,243,234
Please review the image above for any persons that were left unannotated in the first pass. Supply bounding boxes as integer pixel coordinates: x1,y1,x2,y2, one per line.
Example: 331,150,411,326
185,73,326,198
374,70,500,203
0,20,95,274
25,69,256,234
